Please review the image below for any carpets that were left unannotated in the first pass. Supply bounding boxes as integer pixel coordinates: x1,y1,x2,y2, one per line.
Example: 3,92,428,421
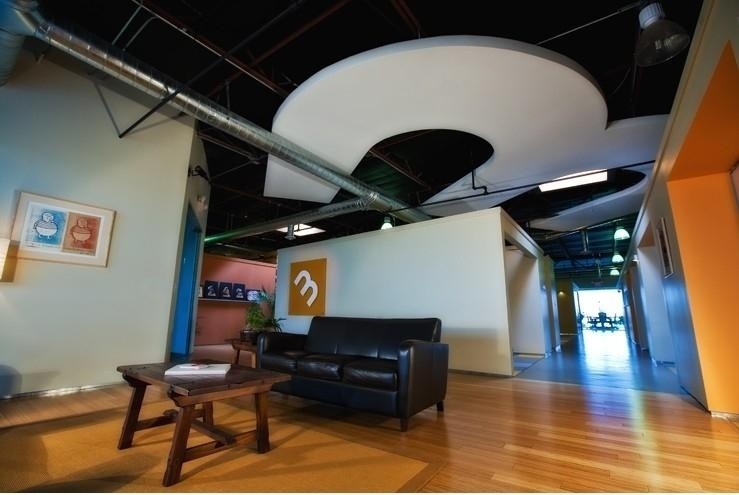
0,398,449,493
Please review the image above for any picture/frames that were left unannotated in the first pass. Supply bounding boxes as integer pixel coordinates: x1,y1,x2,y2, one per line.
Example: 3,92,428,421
654,217,674,279
12,191,117,267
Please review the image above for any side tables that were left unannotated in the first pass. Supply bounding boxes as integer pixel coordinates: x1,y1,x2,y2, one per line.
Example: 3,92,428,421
224,338,260,368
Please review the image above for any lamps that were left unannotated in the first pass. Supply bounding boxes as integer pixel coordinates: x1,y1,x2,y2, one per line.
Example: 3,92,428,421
610,219,630,276
380,211,393,230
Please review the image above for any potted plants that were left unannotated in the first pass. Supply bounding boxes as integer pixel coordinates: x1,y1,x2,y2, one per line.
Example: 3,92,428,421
240,302,286,341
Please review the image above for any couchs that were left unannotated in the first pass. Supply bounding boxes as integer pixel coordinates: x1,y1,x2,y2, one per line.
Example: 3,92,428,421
260,316,449,431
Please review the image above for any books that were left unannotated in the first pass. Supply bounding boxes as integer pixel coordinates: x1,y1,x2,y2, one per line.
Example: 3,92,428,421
164,369,232,380
164,363,233,376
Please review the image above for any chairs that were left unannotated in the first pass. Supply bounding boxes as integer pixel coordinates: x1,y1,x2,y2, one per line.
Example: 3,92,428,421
576,312,624,330
